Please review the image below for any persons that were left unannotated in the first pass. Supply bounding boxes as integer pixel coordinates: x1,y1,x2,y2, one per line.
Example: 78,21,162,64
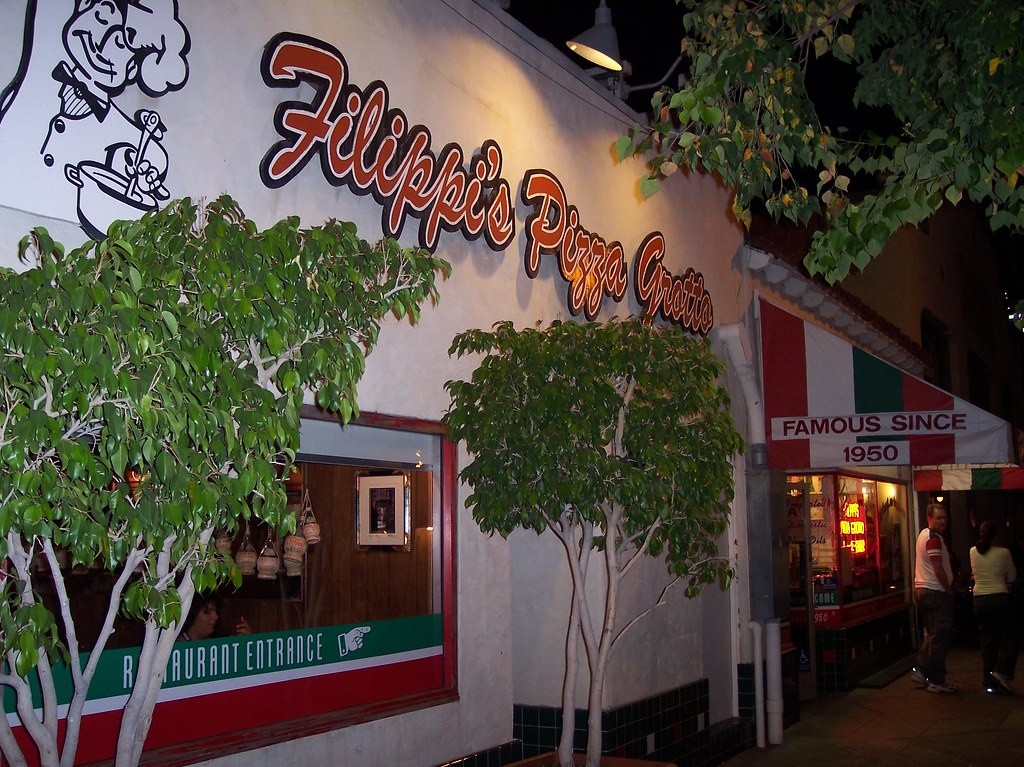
912,505,958,692
967,522,1016,698
174,595,250,641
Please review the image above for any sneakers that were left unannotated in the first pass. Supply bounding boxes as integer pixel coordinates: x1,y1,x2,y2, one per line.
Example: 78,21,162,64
983,671,1015,696
911,663,956,694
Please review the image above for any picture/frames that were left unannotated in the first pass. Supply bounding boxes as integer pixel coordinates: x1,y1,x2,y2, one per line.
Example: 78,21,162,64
351,469,416,554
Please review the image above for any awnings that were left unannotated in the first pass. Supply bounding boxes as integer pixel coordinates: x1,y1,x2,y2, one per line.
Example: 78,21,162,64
761,300,1024,491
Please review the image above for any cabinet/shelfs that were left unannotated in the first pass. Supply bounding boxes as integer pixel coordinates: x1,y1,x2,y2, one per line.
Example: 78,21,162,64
784,465,917,697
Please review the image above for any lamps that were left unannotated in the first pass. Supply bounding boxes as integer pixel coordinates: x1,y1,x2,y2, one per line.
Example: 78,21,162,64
563,0,624,72
